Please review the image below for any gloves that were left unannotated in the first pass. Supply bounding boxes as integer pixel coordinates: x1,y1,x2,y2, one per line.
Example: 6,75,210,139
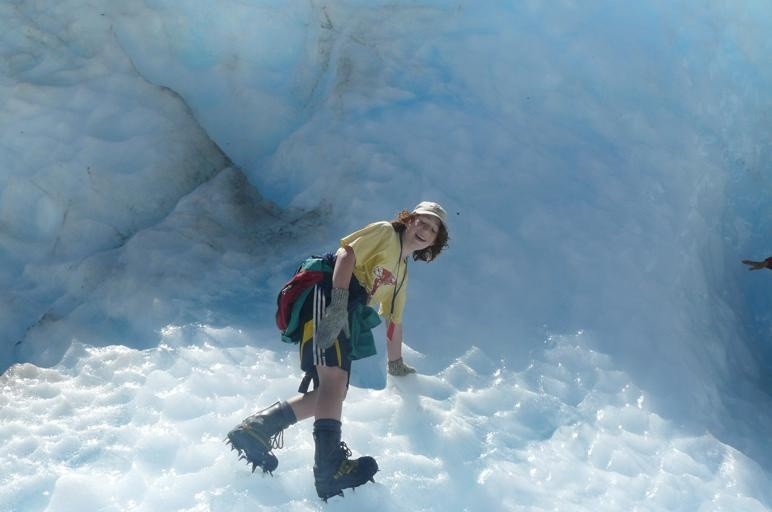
388,358,415,376
316,288,350,349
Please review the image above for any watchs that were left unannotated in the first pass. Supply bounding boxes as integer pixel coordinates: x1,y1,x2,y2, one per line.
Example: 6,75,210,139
224,201,450,503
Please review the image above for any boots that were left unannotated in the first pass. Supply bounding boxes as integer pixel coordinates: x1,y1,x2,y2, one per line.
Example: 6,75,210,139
224,400,297,477
313,418,378,502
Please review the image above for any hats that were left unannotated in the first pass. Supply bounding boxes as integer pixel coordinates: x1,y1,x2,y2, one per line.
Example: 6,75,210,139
412,201,447,222
